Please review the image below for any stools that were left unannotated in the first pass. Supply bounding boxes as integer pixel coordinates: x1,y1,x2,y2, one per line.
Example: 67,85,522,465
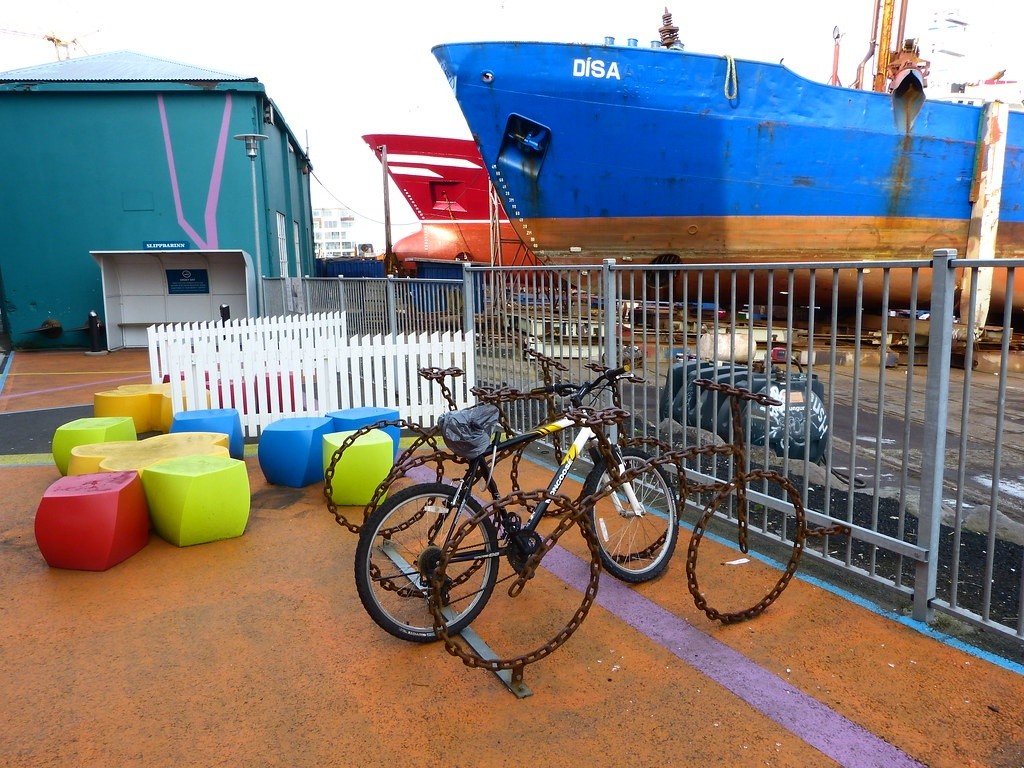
35,370,250,571
257,407,401,506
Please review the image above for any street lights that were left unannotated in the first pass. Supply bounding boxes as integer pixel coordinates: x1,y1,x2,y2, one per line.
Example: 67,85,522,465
232,133,271,322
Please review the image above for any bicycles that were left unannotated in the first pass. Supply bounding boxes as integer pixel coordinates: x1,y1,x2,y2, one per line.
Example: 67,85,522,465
321,343,594,539
349,362,680,644
424,376,809,671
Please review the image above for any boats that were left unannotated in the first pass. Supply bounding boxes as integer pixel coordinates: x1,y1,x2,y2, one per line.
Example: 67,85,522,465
428,0,1024,318
358,130,562,291
